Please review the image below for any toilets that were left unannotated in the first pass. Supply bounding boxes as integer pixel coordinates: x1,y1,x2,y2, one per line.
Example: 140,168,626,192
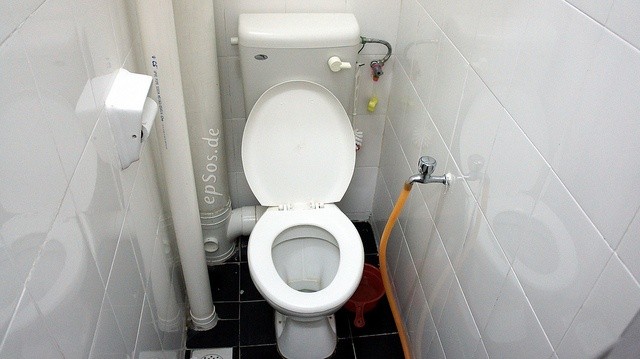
229,12,366,358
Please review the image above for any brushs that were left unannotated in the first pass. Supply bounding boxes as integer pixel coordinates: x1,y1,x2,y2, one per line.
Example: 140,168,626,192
352,63,363,150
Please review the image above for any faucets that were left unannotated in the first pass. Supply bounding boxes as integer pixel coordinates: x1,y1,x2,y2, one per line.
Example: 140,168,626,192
403,155,450,192
